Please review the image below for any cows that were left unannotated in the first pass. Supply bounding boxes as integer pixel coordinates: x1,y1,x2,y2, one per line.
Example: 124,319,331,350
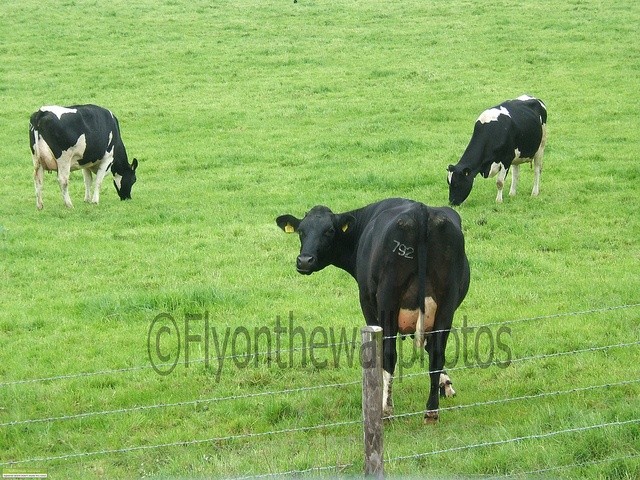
276,198,470,425
446,94,547,207
29,104,138,210
